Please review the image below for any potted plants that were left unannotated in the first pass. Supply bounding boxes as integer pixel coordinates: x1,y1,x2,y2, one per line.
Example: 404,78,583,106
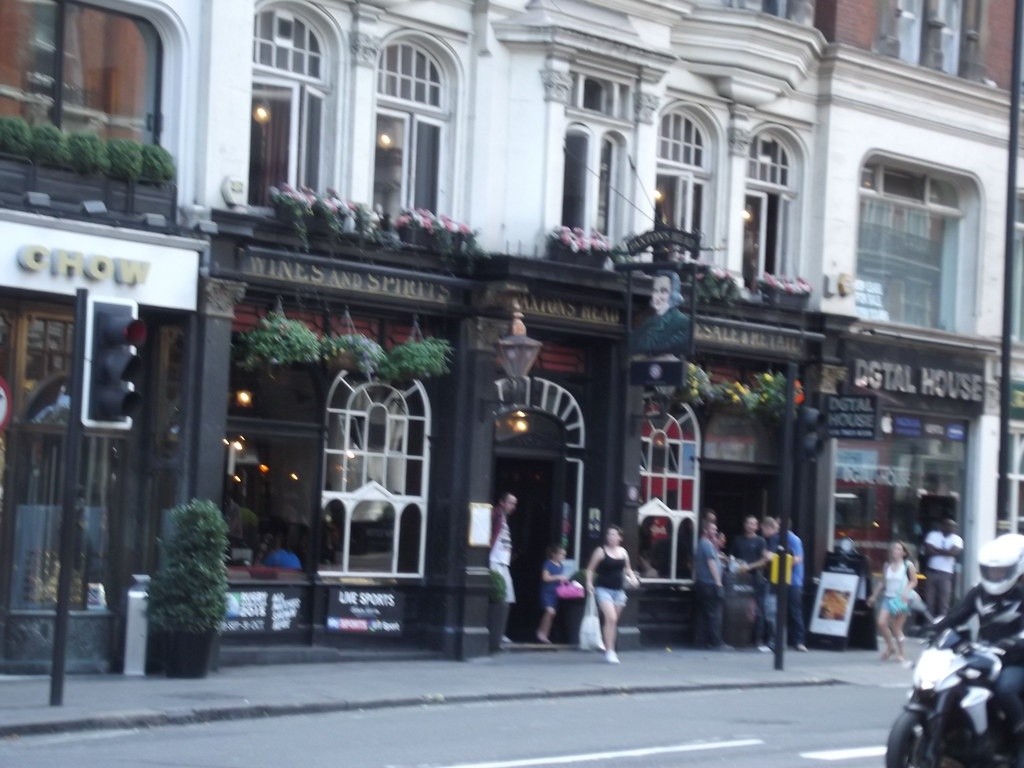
143,498,231,680
396,334,453,380
0,104,180,221
322,329,392,373
245,311,322,368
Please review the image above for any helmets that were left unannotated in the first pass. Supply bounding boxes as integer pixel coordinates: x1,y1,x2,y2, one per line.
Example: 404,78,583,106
976,533,1024,595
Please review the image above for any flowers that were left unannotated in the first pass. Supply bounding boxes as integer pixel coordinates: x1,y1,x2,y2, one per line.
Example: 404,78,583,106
688,360,810,414
696,266,742,309
763,268,814,294
267,182,493,273
552,224,614,252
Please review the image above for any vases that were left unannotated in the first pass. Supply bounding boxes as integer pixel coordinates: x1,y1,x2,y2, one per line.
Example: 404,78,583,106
398,225,463,250
551,236,608,266
273,201,324,226
760,282,811,307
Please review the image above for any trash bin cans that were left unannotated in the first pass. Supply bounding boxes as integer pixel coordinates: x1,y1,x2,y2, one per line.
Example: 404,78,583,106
124,574,153,676
723,571,758,648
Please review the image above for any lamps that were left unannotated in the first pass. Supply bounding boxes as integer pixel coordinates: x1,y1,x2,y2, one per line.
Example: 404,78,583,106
476,298,544,420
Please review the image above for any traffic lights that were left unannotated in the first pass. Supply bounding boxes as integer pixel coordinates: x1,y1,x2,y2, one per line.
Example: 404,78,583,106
74,287,140,438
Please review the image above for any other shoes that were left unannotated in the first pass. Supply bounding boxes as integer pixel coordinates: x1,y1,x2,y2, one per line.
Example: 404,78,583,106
537,631,551,646
757,644,771,654
603,648,620,666
714,641,735,652
883,647,895,663
796,643,807,652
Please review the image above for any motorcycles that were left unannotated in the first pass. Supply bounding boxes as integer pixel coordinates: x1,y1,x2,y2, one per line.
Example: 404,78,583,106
884,624,1023,768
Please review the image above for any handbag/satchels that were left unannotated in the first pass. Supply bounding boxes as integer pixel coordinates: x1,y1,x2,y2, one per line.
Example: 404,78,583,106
579,590,606,651
555,578,584,600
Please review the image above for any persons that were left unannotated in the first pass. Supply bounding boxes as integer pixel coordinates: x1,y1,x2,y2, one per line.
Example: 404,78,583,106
924,517,964,619
866,541,917,661
225,491,260,548
52,505,99,565
696,509,735,650
253,535,303,571
934,532,1024,768
732,515,808,652
537,544,570,644
639,550,668,578
488,493,516,643
586,525,638,662
